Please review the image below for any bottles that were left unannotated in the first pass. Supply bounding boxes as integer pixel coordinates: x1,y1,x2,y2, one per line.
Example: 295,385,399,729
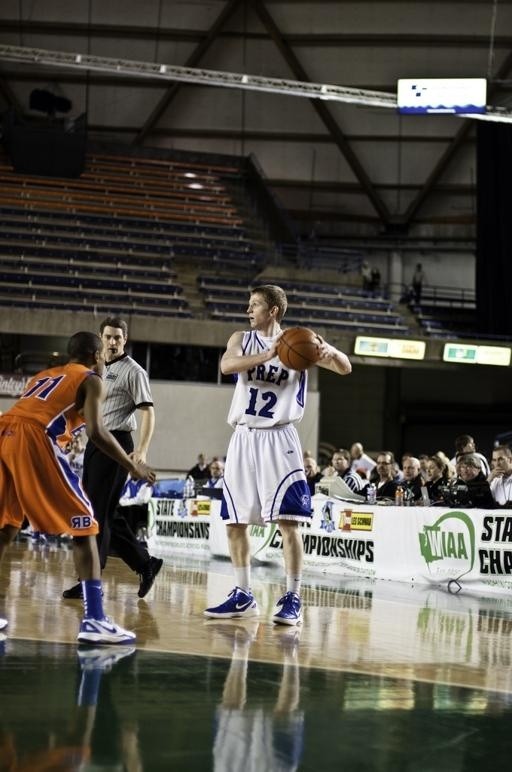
184,474,195,497
395,486,404,505
368,482,376,504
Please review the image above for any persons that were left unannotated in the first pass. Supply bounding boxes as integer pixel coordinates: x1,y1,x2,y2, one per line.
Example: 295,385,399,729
203,283,353,628
186,432,512,509
2,330,157,646
62,315,163,599
359,259,427,307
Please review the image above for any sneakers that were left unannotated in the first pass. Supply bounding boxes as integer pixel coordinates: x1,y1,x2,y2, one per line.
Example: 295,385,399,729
137,599,159,639
138,556,163,598
205,619,259,642
273,591,303,626
77,615,137,644
0,632,7,642
273,626,303,647
0,618,8,629
203,586,258,619
77,645,136,672
63,578,83,599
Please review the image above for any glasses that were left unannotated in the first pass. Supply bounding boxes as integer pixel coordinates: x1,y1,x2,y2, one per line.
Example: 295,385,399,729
376,462,391,466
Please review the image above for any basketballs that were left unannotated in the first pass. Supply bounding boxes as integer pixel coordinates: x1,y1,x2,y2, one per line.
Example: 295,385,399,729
277,327,321,370
